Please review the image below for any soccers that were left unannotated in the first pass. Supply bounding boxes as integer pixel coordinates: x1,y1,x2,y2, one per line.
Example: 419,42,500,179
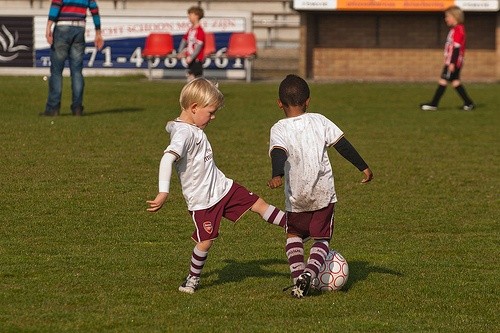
307,248,349,292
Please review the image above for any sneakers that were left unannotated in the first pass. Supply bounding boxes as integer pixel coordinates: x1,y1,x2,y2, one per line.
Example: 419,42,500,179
291,271,311,299
422,104,438,111
179,274,201,294
464,105,473,111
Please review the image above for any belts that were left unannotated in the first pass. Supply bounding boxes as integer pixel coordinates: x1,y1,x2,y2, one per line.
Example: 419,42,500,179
57,21,85,27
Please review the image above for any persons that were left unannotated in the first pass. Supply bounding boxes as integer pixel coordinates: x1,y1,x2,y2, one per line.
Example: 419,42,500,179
177,6,205,84
419,5,476,110
269,74,374,299
38,0,104,115
146,77,311,294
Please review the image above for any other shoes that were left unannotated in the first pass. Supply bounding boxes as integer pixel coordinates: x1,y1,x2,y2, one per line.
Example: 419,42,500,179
74,108,82,115
39,111,59,116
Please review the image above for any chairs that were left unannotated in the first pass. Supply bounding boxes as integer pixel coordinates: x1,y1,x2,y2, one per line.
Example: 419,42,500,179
187,33,216,80
226,33,258,82
142,34,174,80
